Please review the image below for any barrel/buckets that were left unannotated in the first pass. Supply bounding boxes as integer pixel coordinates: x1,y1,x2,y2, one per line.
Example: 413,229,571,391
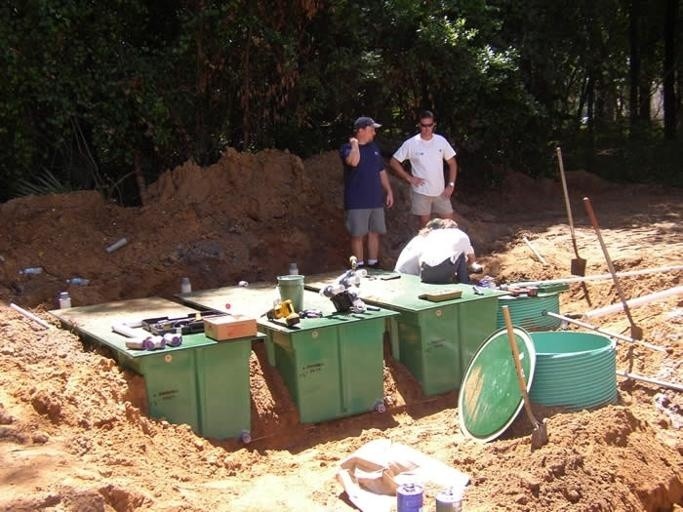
181,277,192,297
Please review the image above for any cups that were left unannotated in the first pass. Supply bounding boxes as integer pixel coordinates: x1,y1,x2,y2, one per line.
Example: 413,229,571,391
431,490,463,512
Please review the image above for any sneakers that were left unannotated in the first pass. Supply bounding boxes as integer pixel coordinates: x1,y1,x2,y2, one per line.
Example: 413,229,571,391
369,261,384,268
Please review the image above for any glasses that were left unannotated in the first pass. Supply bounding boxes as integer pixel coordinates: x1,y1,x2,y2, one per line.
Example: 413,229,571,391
420,121,434,127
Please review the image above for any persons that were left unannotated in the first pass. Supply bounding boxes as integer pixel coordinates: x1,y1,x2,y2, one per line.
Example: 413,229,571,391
416,218,476,285
392,216,444,278
386,110,459,231
337,115,394,269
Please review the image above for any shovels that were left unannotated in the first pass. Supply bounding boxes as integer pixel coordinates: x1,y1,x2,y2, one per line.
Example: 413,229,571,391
583,197,643,341
501,305,549,453
557,147,587,277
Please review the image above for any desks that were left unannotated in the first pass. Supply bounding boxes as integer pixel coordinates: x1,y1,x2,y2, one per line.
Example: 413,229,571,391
49,293,268,445
174,281,401,423
302,268,512,398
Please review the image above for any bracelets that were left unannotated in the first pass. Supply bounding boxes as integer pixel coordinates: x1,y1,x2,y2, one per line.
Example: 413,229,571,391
448,181,455,187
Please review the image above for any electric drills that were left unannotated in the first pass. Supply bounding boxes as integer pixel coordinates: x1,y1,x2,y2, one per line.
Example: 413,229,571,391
267,299,300,327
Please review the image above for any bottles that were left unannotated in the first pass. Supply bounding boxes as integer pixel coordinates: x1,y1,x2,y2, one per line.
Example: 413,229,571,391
106,238,129,255
396,481,426,512
181,277,193,294
18,266,44,275
58,290,72,309
65,278,91,286
288,262,299,275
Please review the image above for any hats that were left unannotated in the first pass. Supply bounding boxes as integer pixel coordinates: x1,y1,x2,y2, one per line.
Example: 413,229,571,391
354,117,382,128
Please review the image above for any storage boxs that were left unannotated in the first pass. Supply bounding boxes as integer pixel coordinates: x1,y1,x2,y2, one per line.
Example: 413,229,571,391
201,312,258,342
